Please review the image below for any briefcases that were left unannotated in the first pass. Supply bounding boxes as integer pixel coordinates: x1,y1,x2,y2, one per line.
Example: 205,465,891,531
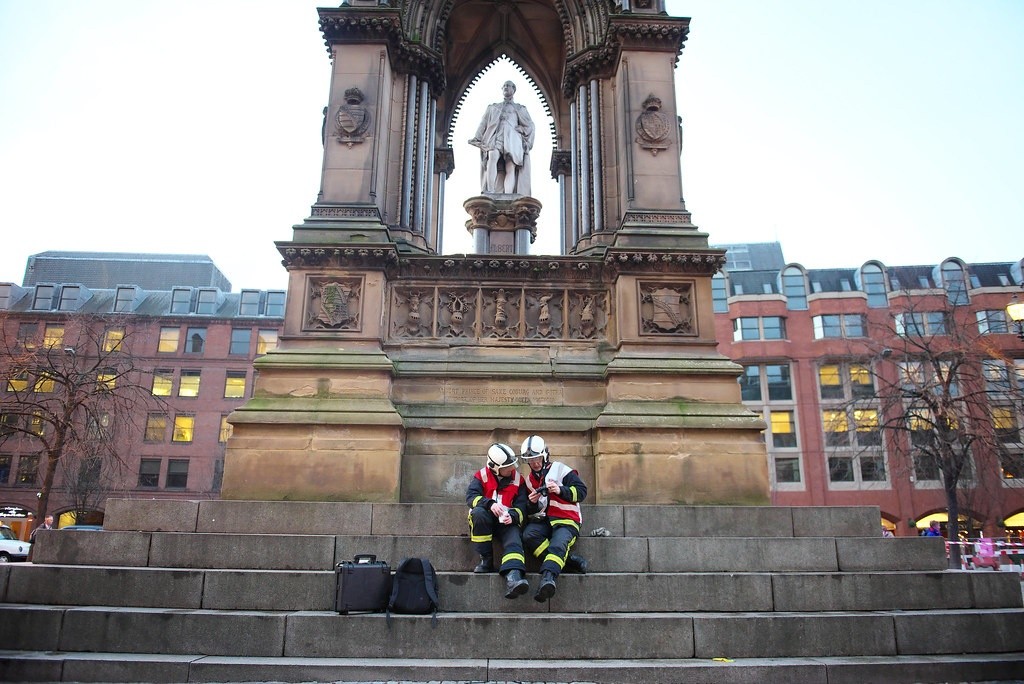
334,554,394,615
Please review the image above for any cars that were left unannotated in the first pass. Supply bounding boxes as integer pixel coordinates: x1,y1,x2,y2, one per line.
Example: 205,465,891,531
0,525,32,563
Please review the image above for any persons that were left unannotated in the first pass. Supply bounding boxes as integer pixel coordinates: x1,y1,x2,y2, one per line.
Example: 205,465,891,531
31,514,53,544
467,80,536,195
520,434,587,603
882,526,895,537
920,520,942,536
463,442,530,599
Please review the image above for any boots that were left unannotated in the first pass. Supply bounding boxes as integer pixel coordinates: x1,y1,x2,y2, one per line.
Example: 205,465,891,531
534,569,557,603
569,554,589,574
504,569,530,600
474,552,495,573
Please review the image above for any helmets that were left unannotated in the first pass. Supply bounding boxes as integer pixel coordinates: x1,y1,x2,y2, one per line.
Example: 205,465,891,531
520,435,550,464
486,443,519,476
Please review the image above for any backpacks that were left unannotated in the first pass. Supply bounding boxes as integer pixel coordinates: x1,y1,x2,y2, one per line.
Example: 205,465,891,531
386,558,444,626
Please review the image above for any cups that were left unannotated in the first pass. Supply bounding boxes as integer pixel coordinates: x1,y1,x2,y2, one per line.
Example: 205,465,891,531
498,506,509,523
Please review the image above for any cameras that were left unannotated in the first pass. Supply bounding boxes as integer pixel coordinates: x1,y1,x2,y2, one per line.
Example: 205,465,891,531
536,486,549,497
